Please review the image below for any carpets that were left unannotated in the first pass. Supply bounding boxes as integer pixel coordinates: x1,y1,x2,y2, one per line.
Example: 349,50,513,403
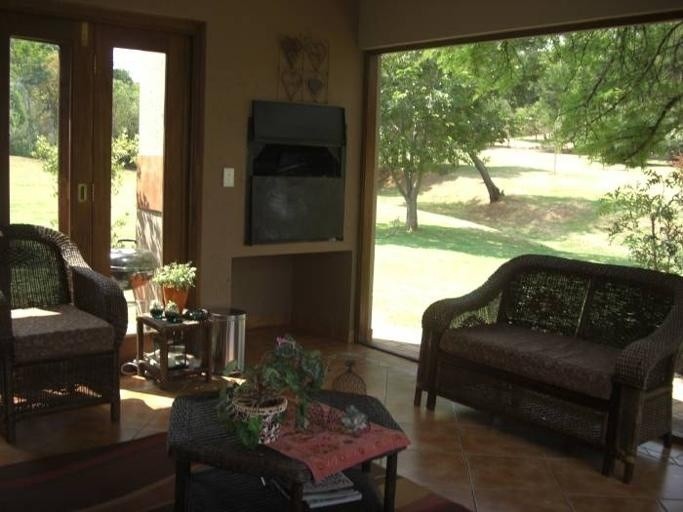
0,431,475,512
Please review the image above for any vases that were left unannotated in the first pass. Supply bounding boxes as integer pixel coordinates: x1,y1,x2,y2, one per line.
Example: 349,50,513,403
230,390,289,442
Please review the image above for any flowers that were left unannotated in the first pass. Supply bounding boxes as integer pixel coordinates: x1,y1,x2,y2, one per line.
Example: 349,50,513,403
215,333,335,446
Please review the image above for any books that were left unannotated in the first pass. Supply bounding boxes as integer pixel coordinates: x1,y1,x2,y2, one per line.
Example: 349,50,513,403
271,468,362,509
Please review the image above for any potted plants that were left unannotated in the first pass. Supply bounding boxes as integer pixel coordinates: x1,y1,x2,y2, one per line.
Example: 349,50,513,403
149,259,197,313
164,300,179,321
147,299,162,319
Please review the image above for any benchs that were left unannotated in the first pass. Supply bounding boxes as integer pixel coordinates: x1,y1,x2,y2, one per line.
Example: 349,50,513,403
413,253,683,481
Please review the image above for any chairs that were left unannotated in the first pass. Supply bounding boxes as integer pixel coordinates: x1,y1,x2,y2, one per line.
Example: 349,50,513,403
1,222,125,444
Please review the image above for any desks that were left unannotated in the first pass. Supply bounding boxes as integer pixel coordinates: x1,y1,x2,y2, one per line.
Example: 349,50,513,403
153,383,410,511
133,310,214,389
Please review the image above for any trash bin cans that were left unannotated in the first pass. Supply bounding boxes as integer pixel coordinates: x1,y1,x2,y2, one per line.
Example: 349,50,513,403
210,308,246,375
